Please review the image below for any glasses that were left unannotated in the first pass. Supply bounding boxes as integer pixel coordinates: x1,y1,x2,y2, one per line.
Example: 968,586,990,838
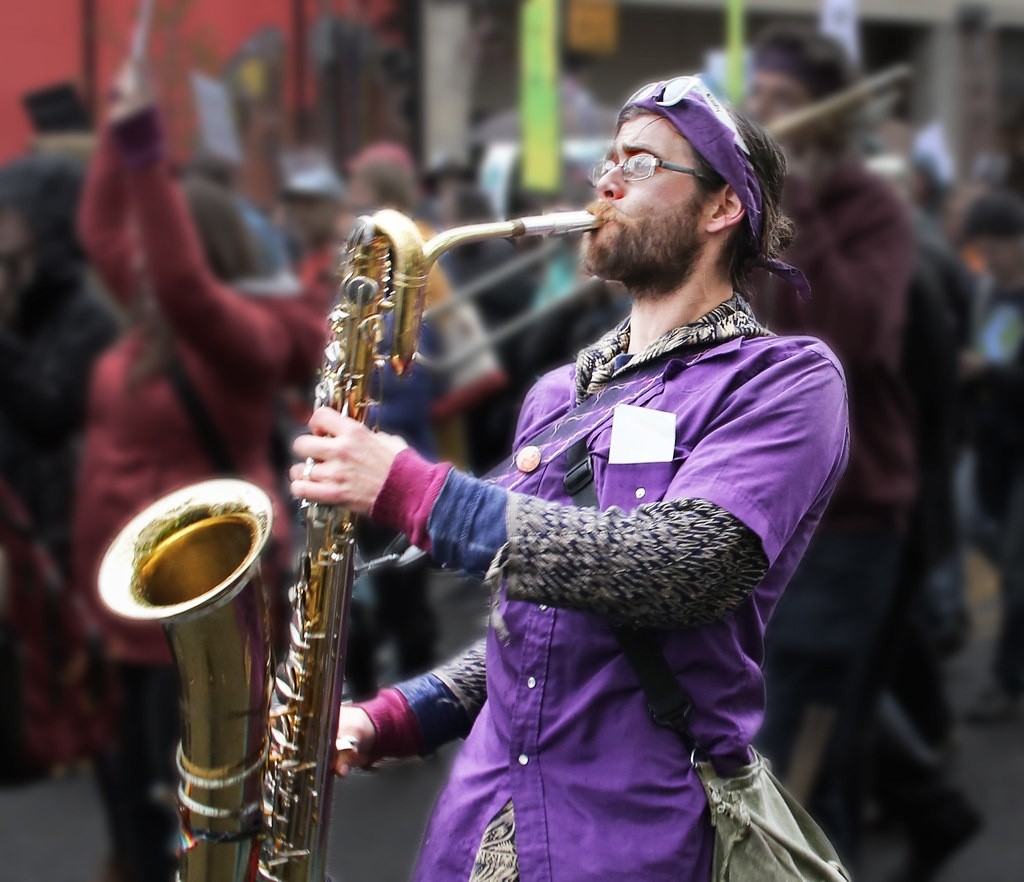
590,153,718,187
626,76,755,162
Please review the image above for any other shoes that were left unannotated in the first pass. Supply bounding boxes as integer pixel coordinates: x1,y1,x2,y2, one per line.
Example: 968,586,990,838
896,792,989,882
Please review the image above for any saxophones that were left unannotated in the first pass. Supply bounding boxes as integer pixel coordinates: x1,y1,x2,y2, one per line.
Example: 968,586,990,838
90,211,608,882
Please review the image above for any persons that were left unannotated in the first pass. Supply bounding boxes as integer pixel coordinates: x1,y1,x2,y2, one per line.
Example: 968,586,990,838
291,78,853,882
0,23,1023,882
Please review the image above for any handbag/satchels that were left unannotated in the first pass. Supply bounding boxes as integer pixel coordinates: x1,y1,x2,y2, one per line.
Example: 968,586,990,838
690,745,852,881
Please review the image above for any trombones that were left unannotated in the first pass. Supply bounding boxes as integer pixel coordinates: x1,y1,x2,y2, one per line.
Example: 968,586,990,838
396,60,919,374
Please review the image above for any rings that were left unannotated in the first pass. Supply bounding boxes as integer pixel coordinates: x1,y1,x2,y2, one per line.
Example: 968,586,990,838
302,456,314,480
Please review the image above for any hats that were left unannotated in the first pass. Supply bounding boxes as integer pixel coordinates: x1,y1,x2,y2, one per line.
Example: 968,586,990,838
284,169,338,202
